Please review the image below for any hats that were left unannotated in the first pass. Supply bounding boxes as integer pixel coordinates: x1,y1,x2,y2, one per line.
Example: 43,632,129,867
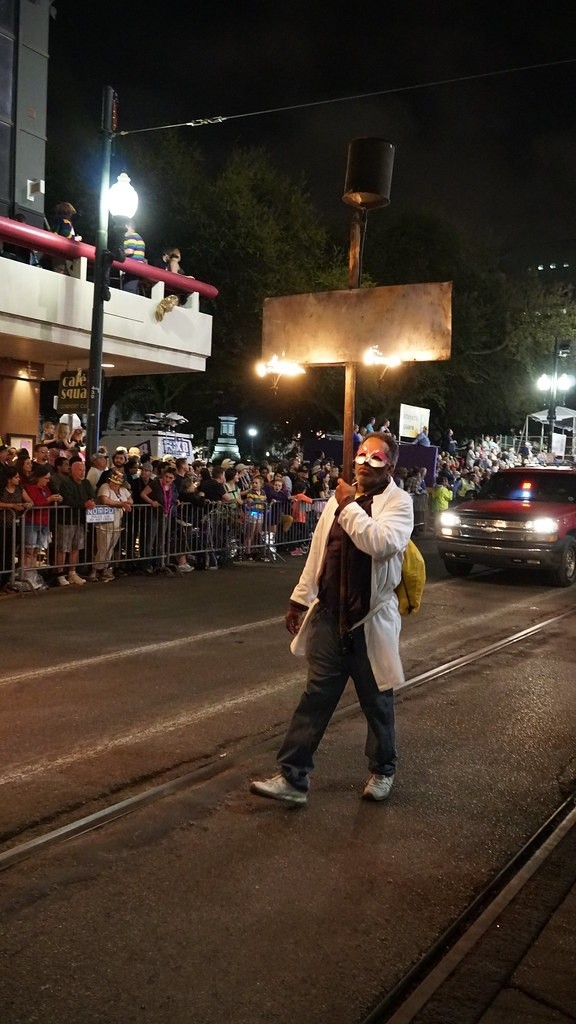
107,471,125,485
138,463,153,471
90,453,109,462
235,463,249,472
222,458,235,465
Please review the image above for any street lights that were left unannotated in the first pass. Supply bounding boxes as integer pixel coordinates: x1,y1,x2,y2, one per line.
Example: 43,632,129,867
84,166,139,479
538,336,575,454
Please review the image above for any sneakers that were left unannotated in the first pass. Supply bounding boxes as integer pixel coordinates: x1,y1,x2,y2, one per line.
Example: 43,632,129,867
68,574,87,584
361,773,394,800
57,575,70,585
249,771,307,804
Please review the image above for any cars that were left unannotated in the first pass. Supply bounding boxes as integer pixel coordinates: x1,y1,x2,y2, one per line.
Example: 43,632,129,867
437,462,576,589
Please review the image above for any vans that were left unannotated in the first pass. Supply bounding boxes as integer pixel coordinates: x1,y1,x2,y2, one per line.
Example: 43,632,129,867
99,412,195,466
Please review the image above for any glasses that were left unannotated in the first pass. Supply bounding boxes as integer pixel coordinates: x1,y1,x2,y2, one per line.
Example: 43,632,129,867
36,450,51,453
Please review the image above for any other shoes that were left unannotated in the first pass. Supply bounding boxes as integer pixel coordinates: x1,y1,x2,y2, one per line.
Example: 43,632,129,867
247,556,255,563
115,569,128,578
90,576,101,582
291,547,304,556
177,563,195,573
99,570,115,581
144,568,157,577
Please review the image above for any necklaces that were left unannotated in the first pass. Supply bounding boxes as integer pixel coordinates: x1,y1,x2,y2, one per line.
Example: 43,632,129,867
114,490,123,502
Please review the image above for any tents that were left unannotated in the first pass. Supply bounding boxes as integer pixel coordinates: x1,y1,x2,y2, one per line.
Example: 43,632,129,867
526,406,576,459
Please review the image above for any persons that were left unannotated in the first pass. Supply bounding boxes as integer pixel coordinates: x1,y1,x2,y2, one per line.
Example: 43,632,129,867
162,243,195,305
248,429,414,801
123,219,145,296
0,418,154,591
266,417,392,556
50,202,77,274
412,425,504,537
505,435,568,470
155,452,292,574
2,212,38,264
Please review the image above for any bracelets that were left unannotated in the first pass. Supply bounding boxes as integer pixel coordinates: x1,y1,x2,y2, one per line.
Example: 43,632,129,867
288,607,302,616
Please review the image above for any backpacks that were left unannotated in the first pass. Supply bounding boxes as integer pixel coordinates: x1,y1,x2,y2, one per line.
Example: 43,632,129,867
393,538,427,616
